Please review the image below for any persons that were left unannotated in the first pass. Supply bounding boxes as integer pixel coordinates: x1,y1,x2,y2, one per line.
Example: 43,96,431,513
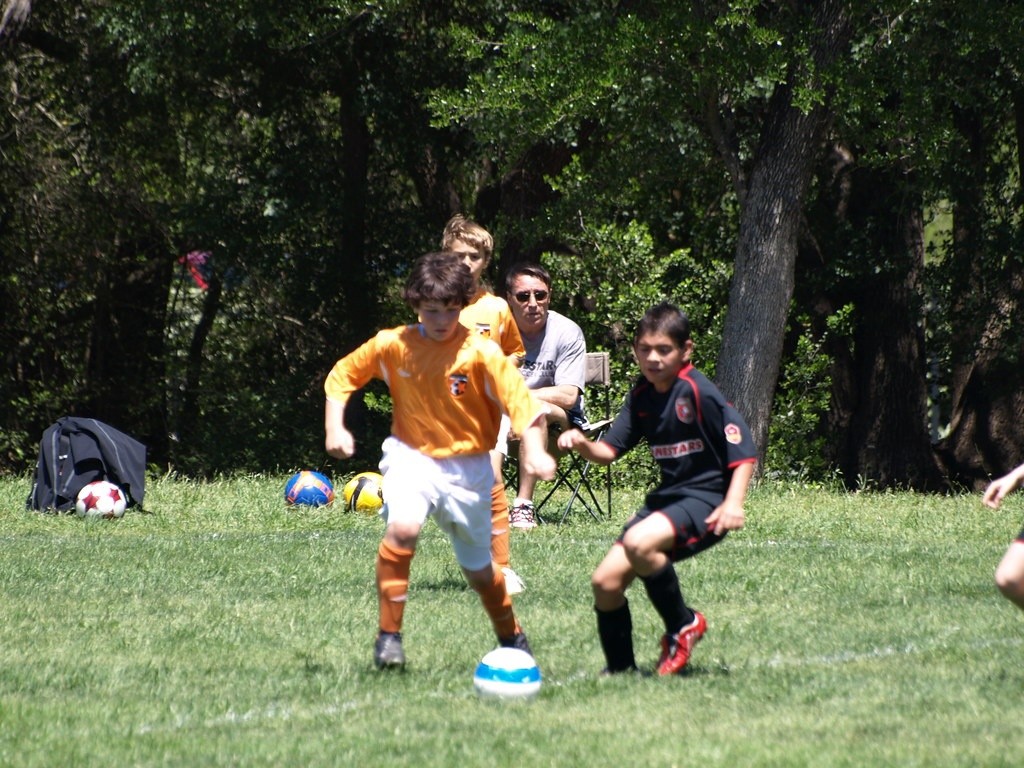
499,262,589,530
556,303,759,677
983,464,1024,610
324,250,555,669
437,213,527,594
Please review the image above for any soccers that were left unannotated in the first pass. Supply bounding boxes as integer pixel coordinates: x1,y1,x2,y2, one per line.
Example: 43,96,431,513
76,479,127,520
343,472,385,516
474,648,541,698
284,470,335,508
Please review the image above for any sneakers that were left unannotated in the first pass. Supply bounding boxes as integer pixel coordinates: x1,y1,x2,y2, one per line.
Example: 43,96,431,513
656,609,708,676
508,497,537,528
374,632,406,671
598,665,640,677
499,632,533,657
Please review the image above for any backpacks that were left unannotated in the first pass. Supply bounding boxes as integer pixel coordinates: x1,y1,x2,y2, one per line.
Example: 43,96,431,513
26,416,78,514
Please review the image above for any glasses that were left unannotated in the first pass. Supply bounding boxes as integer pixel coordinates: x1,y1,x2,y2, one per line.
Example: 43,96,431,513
510,290,549,302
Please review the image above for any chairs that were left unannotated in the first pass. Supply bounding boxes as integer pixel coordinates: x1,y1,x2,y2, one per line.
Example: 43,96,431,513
502,350,612,528
